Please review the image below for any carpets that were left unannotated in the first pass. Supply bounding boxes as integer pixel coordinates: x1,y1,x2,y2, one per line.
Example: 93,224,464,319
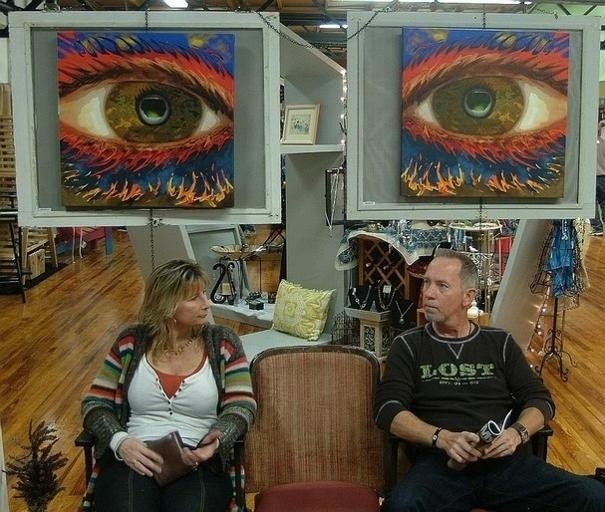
0,260,68,295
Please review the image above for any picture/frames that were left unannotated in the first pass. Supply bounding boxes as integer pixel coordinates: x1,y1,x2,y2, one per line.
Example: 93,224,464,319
324,166,344,226
280,102,321,145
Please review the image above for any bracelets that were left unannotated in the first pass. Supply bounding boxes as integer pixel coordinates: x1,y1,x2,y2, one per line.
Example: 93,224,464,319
510,422,530,443
432,427,443,448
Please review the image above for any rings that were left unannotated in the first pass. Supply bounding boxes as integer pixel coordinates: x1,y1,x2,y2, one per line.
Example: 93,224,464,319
192,462,198,470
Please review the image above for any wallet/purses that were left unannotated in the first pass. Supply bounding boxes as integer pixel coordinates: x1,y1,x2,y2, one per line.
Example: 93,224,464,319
147,430,198,487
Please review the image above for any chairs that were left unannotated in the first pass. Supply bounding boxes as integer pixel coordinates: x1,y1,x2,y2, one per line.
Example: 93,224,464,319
393,424,553,511
245,345,393,511
75,428,244,510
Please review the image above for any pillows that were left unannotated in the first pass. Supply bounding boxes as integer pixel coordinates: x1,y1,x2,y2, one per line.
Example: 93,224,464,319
271,278,337,342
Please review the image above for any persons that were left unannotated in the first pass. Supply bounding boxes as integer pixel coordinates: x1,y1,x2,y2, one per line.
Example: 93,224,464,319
81,258,257,512
373,247,605,512
589,124,605,236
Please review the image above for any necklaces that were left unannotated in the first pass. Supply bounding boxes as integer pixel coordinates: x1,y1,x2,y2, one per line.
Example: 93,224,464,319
164,338,195,357
468,322,474,335
